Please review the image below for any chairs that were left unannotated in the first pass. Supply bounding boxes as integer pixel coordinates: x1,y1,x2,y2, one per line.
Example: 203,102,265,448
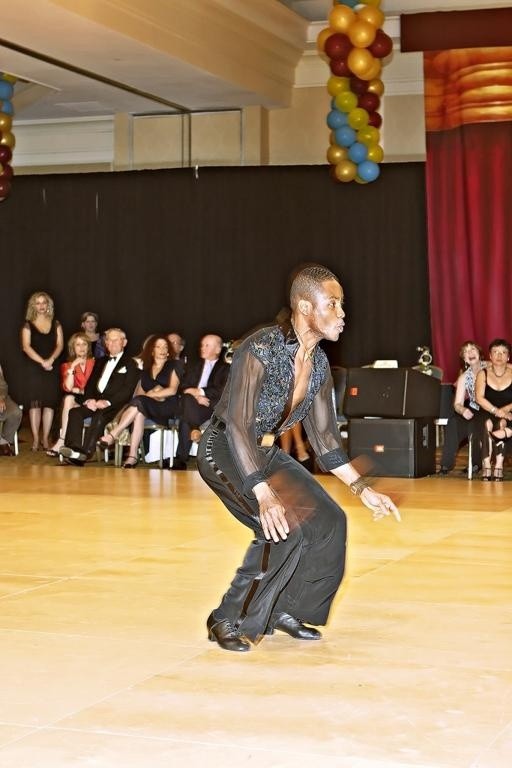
432,384,473,480
13,405,198,469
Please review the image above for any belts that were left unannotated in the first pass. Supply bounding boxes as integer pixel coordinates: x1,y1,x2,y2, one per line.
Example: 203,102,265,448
210,414,275,447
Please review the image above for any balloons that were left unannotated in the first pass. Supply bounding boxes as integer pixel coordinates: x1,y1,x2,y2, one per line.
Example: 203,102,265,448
316,1,395,184
0,69,20,202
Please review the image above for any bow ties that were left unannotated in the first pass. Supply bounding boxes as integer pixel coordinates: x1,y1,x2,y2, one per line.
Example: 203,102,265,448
108,356,116,360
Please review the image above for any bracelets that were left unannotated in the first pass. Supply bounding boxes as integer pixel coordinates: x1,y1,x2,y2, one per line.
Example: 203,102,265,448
349,477,369,496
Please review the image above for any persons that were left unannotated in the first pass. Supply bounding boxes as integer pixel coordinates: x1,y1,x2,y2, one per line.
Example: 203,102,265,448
194,264,403,651
435,340,511,476
171,336,245,470
97,329,191,472
17,291,140,465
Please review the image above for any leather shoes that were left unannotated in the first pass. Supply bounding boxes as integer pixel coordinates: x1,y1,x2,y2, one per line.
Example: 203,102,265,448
206,608,251,651
95,433,114,447
191,428,202,443
263,611,321,639
122,456,138,468
488,428,512,442
58,446,87,465
0,443,15,455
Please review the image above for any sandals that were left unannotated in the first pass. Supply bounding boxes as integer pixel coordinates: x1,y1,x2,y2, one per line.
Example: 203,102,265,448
45,437,64,456
482,467,505,481
